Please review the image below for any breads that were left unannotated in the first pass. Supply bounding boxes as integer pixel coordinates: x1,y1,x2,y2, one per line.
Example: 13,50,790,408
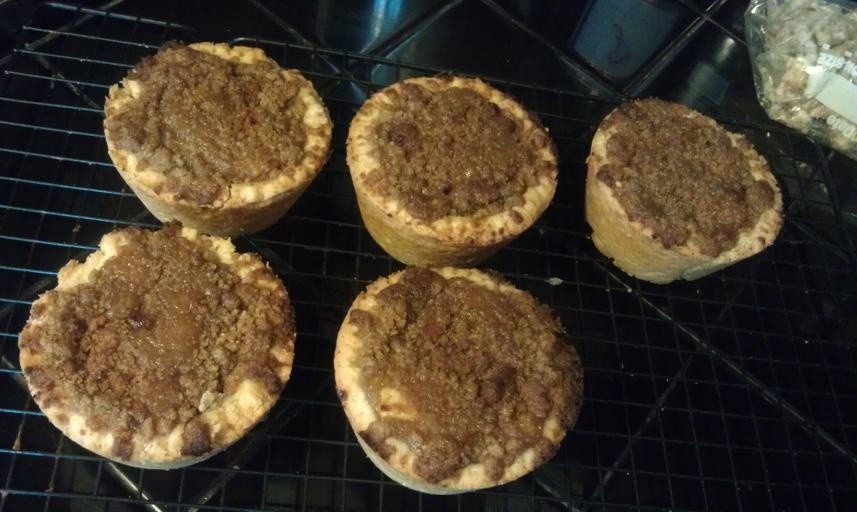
588,97,783,285
332,264,585,496
17,220,298,468
102,41,336,236
346,76,560,267
